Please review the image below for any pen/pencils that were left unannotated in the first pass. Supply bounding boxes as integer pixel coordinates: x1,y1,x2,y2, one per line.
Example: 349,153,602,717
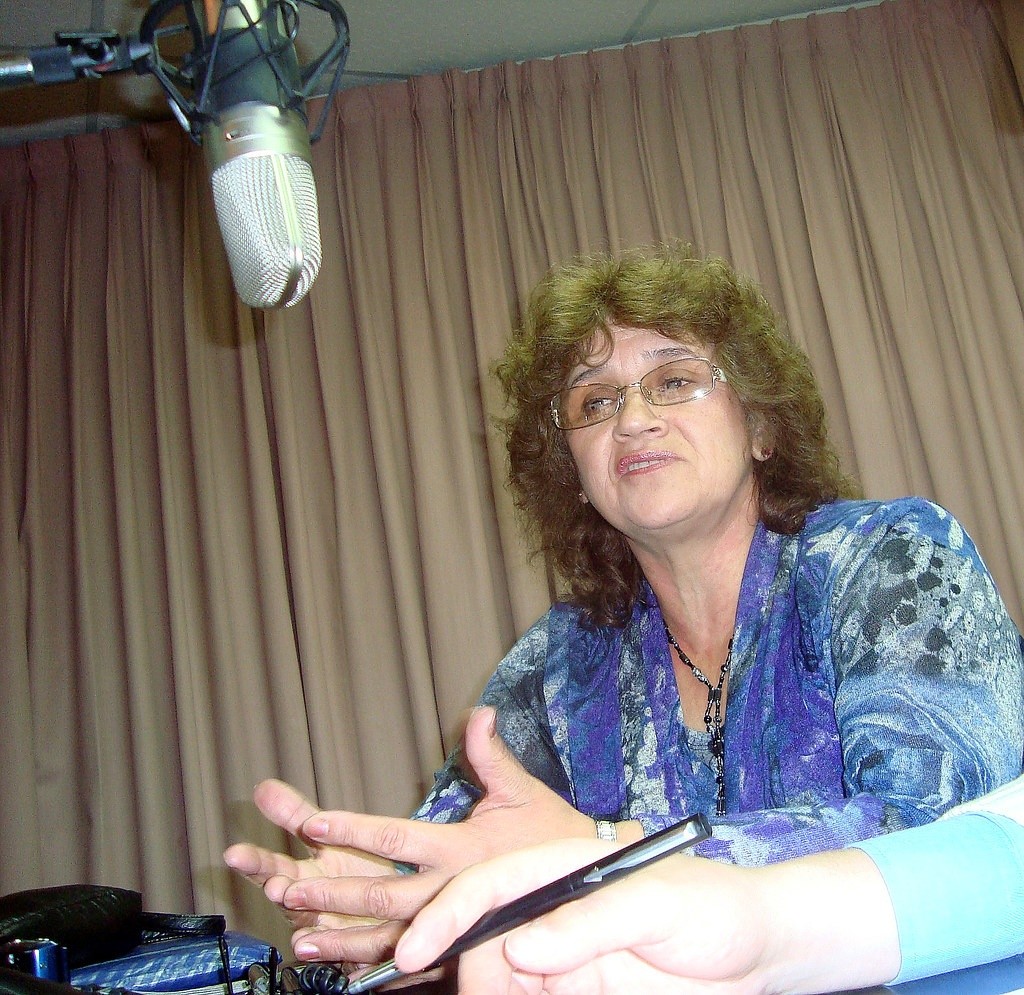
342,812,715,995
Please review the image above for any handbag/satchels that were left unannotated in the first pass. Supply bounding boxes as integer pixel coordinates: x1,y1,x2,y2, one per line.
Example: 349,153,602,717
0,883,227,988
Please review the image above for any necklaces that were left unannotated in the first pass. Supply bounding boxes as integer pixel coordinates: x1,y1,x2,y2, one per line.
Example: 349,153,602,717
665,623,734,815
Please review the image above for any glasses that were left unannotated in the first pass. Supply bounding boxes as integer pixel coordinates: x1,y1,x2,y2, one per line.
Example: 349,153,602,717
549,355,728,432
244,960,303,995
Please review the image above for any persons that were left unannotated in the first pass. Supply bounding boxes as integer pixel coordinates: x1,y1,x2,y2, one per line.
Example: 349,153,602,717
223,252,1024,994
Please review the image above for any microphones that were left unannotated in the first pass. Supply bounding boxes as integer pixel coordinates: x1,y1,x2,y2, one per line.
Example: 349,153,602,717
195,0,322,310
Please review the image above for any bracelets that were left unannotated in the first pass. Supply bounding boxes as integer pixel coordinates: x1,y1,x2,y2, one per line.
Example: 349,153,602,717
598,821,617,841
